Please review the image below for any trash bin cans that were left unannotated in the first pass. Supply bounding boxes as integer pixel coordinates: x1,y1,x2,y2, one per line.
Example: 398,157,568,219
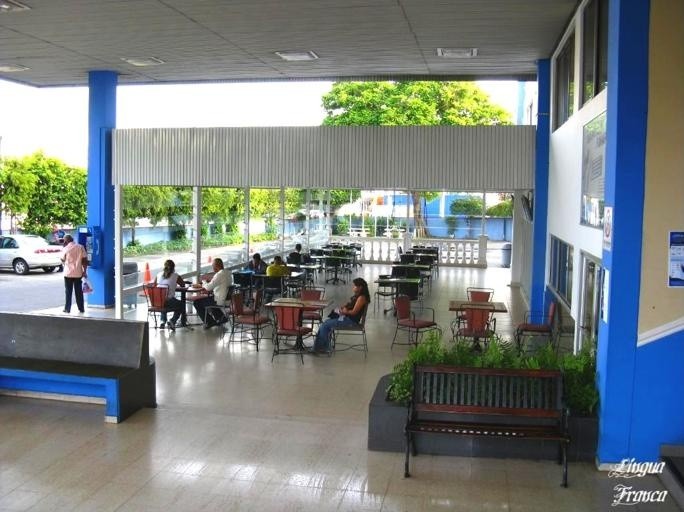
502,243,513,268
115,262,138,309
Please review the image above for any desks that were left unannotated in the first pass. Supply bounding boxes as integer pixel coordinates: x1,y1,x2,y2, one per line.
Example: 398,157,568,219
448,299,508,328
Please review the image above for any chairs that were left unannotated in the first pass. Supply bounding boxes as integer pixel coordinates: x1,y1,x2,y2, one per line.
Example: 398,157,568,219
140,245,440,365
465,287,494,304
514,301,556,357
453,304,496,346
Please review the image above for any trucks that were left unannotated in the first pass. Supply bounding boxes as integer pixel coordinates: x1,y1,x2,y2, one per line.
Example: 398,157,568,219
335,191,413,218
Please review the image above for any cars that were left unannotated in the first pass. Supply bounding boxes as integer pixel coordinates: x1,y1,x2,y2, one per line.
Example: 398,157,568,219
1,235,64,274
274,209,322,241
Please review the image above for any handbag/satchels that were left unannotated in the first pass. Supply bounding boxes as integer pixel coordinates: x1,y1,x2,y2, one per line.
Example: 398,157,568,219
327,308,339,320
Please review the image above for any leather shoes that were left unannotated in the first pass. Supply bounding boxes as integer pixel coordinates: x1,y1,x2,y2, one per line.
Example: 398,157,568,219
204,317,228,329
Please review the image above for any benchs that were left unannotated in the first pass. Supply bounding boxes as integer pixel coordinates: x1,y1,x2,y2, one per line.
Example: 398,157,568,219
0,313,155,425
404,362,571,487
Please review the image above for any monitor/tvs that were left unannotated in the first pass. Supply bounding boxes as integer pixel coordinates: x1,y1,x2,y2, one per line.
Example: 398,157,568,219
520,197,532,221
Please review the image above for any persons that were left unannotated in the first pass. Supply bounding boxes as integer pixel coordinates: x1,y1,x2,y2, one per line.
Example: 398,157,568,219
266,257,291,304
192,259,233,330
309,278,370,354
288,243,302,265
247,253,268,275
154,259,185,332
60,235,87,313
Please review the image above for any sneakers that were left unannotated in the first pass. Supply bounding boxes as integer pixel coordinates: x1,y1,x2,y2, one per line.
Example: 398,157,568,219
166,320,175,331
159,321,165,329
